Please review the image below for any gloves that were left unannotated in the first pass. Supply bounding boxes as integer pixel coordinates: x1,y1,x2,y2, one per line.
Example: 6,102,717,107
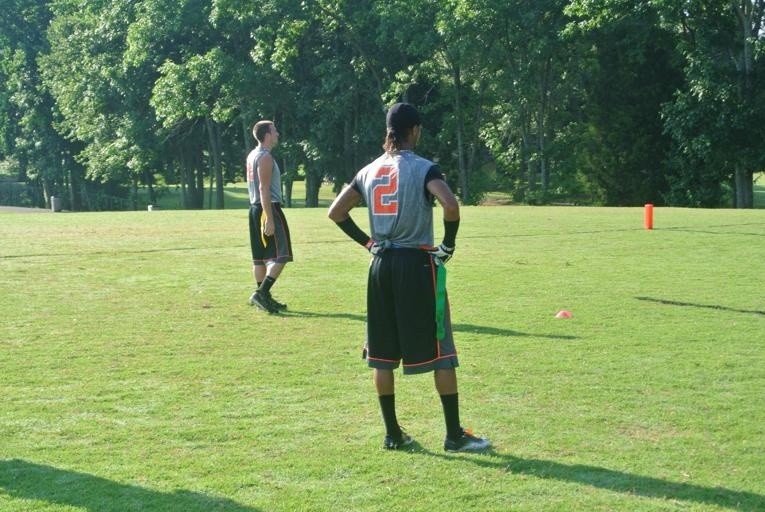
426,242,455,267
364,239,384,256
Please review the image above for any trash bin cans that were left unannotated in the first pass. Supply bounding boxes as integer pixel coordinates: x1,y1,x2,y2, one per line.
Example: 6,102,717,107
147,204,160,211
50,196,64,212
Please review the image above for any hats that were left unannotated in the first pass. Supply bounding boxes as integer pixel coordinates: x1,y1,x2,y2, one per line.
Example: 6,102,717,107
386,102,426,129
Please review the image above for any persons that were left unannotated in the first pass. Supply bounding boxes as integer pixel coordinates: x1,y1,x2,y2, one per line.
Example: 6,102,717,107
329,103,492,453
245,120,294,315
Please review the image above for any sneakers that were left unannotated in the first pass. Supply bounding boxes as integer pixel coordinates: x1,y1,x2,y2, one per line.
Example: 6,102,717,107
444,429,491,454
257,297,287,310
249,289,279,313
382,426,414,449
